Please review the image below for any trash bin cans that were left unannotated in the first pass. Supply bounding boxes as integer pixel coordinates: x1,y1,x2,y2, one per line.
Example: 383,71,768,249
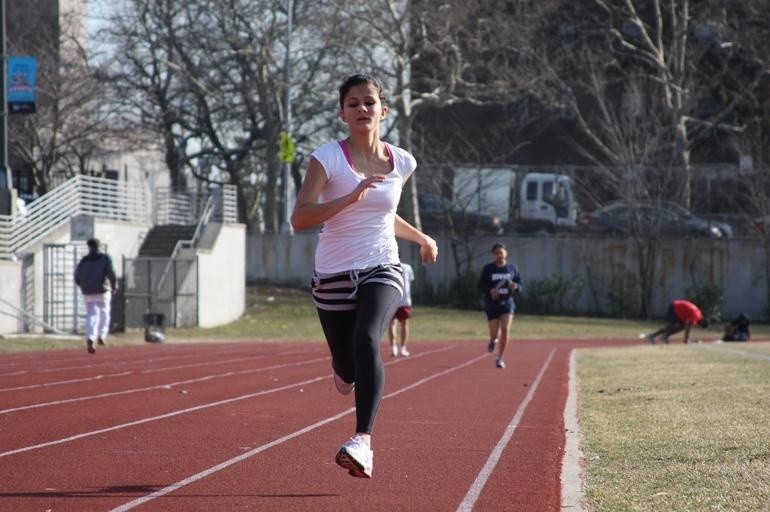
144,313,166,341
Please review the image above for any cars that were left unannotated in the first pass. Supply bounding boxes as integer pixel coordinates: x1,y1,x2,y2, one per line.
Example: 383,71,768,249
397,191,504,236
574,199,733,240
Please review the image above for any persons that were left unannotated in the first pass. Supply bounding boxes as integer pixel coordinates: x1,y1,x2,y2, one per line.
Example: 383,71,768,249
387,249,415,358
286,72,440,480
477,242,523,368
722,311,752,341
643,298,709,346
73,238,119,354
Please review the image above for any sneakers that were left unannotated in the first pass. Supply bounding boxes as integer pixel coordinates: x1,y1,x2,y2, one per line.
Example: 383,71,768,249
390,347,410,357
645,334,671,345
86,338,106,354
487,337,498,353
496,359,505,369
333,369,355,396
334,436,374,480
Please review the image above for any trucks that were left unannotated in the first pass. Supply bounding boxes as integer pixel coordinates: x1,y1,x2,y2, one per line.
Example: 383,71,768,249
452,164,577,240
